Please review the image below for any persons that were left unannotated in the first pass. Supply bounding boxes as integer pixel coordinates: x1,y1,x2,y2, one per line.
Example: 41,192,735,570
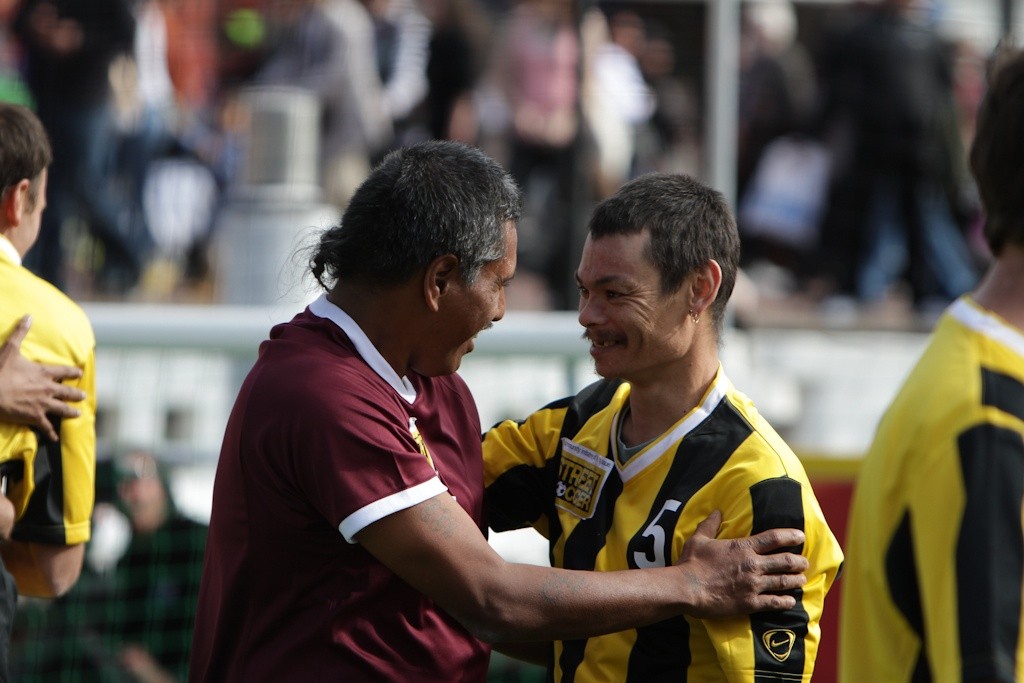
0,0,1024,683
190,139,810,683
